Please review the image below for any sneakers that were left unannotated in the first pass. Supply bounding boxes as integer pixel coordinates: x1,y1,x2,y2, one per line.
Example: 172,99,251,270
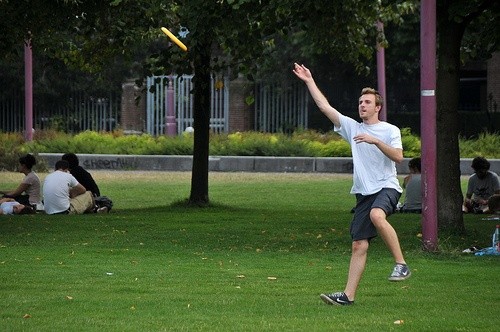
95,206,107,214
388,263,411,281
320,291,354,306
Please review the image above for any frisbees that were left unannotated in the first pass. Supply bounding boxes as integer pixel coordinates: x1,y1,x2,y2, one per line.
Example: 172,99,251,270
161,26,187,52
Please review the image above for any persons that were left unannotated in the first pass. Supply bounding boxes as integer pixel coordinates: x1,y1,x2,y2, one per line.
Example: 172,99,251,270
293,62,412,306
42,160,108,216
0,154,41,210
460,157,500,214
62,153,100,199
398,158,423,212
0,197,37,214
